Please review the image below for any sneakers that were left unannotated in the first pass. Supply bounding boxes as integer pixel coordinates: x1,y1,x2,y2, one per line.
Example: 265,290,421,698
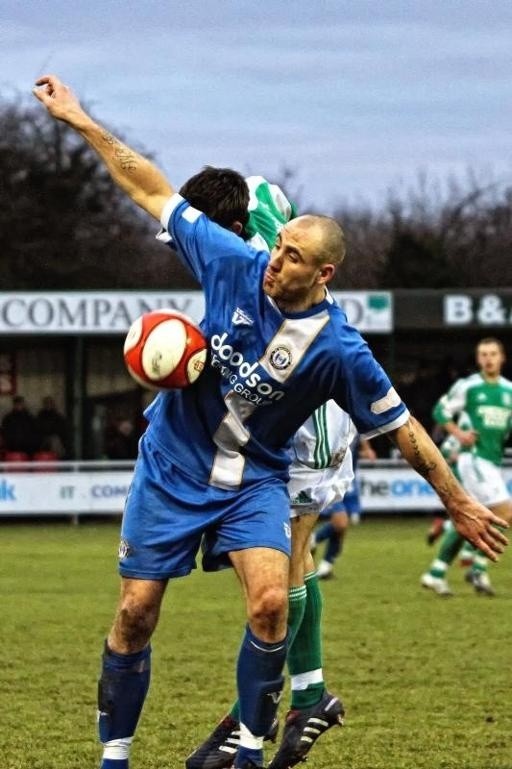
426,517,445,546
464,570,496,596
97,737,131,769
184,684,346,768
316,560,336,580
419,571,453,597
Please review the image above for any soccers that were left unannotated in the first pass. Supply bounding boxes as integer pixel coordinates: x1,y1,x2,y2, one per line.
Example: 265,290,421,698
124,309,209,393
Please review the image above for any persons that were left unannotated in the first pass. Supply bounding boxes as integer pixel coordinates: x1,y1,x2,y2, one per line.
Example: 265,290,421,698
0,386,159,473
31,72,510,769
356,387,459,462
174,165,356,768
418,337,511,598
306,432,378,581
